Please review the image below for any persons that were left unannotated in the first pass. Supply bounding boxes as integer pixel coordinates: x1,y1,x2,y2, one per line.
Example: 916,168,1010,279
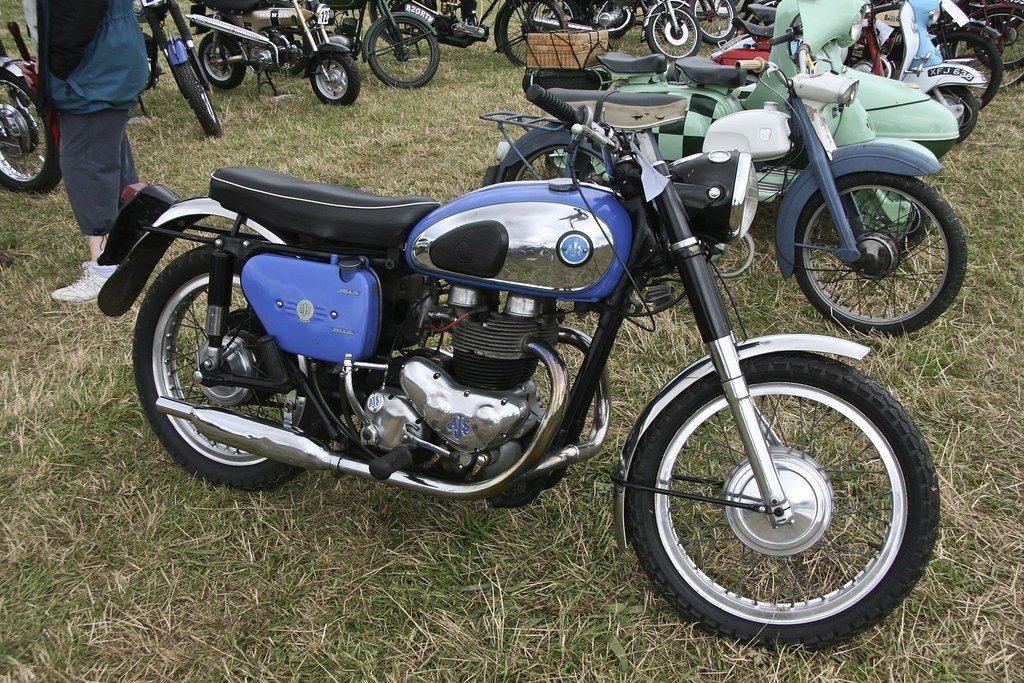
22,0,150,304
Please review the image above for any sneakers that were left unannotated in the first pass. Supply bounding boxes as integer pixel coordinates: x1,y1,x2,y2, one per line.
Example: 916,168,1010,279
50,261,119,306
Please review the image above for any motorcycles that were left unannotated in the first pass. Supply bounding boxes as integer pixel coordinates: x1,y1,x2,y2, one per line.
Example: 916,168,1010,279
478,42,969,338
0,21,62,194
184,0,1024,256
95,74,942,653
131,0,223,139
